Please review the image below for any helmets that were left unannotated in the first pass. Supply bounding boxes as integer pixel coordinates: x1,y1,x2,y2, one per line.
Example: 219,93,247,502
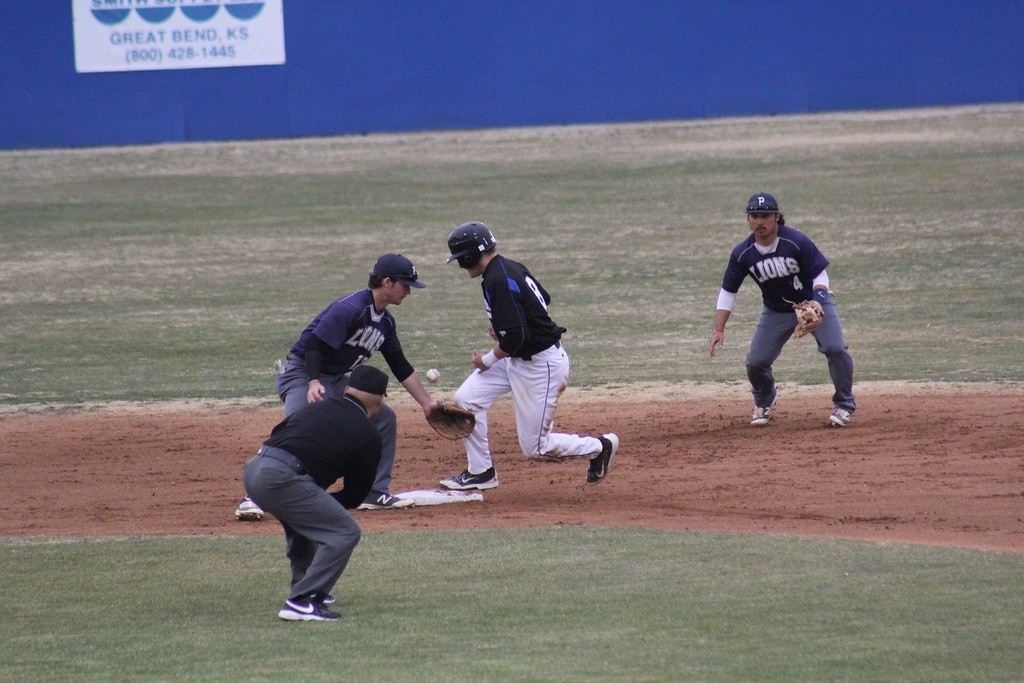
445,221,497,268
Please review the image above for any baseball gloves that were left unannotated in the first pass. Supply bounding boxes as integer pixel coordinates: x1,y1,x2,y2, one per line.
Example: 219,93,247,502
425,397,477,442
779,295,826,340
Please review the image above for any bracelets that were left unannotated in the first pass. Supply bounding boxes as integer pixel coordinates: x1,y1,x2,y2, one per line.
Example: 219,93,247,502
713,329,723,333
482,350,499,367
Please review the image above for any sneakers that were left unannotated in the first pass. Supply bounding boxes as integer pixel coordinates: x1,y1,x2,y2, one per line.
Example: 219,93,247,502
234,494,264,520
357,490,416,510
439,466,499,490
278,597,342,621
830,407,850,426
586,433,619,486
322,594,335,604
751,389,778,426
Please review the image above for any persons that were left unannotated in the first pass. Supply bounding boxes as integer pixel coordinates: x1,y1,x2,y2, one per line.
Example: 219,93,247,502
710,192,857,427
440,222,618,491
243,365,389,622
234,254,476,521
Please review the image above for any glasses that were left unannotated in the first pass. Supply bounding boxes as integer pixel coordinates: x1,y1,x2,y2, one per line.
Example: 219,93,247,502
383,273,418,282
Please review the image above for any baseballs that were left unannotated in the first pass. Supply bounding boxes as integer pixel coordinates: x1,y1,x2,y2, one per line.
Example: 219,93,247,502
425,368,441,383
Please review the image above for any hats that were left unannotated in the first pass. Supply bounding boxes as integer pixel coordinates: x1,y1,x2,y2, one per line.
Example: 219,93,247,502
348,365,389,397
374,253,427,289
745,192,779,213
